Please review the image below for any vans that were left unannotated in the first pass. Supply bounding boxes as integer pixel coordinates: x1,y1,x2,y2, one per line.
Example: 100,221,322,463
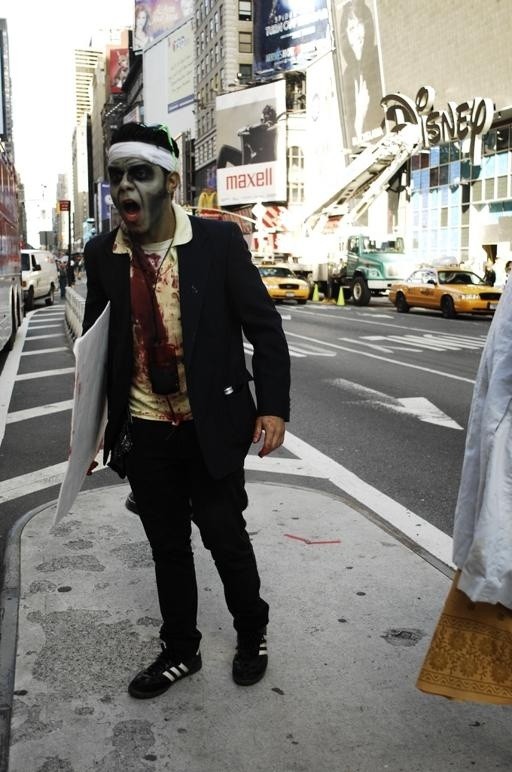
21,249,60,305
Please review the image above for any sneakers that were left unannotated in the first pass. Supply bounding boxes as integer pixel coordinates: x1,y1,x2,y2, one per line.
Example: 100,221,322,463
232,634,269,686
127,649,202,699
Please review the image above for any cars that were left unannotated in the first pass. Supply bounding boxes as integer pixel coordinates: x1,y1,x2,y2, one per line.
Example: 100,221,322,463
388,266,504,318
255,264,311,304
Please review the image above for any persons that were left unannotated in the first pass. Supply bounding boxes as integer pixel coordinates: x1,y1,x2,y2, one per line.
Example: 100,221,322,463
134,4,154,51
82,120,290,698
480,263,495,286
216,105,276,168
339,0,386,145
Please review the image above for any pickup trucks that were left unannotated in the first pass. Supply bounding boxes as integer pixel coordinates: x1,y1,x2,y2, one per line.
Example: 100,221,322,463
328,235,405,305
